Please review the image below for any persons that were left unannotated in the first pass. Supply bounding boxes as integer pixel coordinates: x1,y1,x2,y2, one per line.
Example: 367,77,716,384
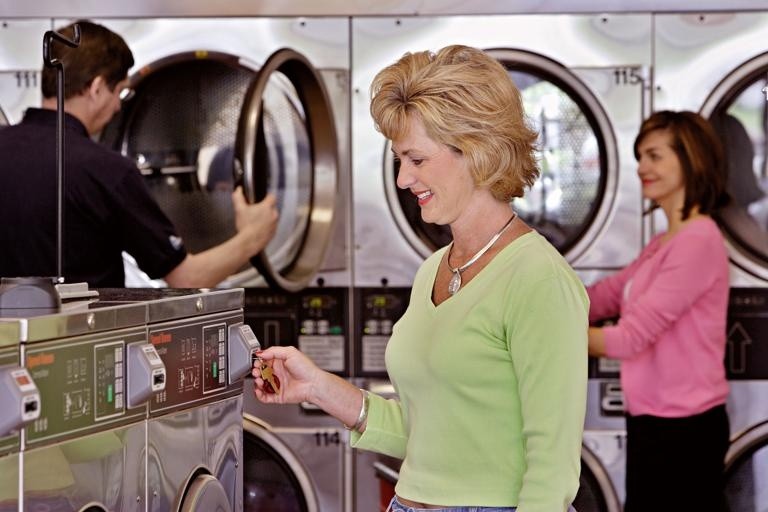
718,112,768,256
251,45,592,512
588,110,730,512
0,21,279,289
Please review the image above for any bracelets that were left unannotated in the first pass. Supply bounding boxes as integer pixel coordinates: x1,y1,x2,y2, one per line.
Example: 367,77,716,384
344,389,369,431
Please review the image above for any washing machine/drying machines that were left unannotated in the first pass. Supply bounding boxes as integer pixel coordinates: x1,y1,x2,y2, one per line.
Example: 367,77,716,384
2,18,349,386
655,11,768,378
349,379,627,512
1,290,243,512
354,15,656,379
619,377,768,512
235,373,349,511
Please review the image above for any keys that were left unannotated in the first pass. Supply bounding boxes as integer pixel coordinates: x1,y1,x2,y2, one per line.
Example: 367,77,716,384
251,351,281,395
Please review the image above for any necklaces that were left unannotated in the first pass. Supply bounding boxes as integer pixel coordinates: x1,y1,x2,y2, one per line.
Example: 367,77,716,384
447,212,519,293
